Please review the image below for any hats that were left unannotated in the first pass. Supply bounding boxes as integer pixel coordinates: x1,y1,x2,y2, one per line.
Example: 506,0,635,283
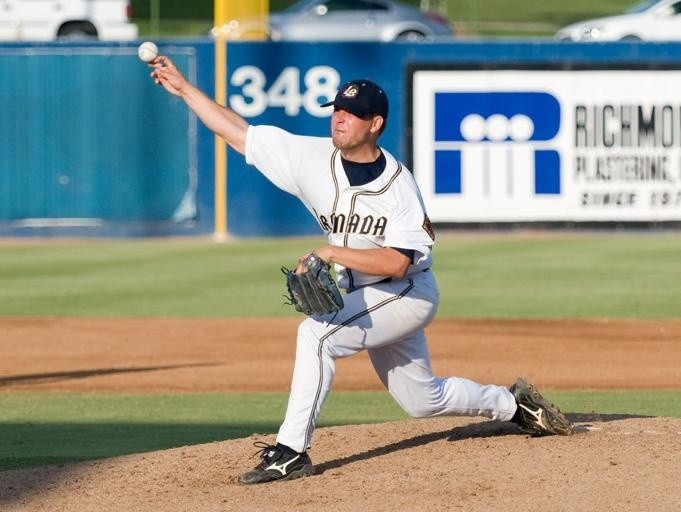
319,79,389,120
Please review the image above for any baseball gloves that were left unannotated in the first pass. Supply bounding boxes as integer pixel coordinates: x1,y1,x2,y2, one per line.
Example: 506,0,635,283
280,253,345,318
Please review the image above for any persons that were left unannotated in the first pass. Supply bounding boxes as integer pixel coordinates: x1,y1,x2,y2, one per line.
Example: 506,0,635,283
148,54,576,486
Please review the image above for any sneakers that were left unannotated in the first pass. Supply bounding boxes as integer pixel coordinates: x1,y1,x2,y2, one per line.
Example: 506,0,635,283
238,444,316,485
508,377,576,437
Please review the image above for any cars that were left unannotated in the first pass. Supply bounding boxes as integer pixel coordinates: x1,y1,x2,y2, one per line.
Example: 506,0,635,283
0,0,141,44
205,0,459,48
550,1,681,44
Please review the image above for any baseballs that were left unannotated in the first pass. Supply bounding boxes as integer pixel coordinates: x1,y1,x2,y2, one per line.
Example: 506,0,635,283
137,41,158,63
